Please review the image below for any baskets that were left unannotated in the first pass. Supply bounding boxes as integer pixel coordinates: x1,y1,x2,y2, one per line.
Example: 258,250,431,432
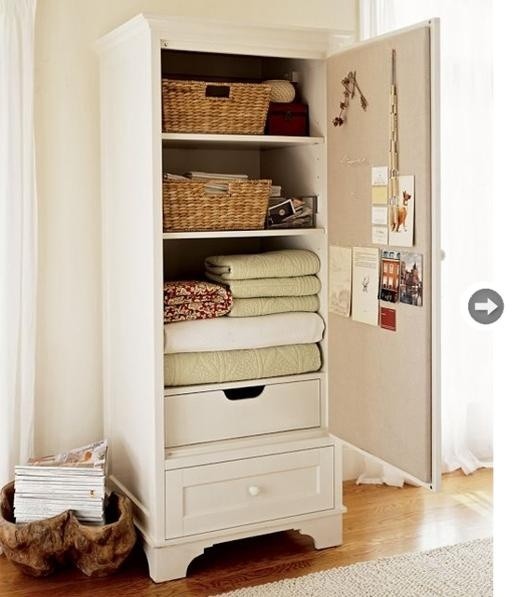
161,79,272,135
162,178,272,232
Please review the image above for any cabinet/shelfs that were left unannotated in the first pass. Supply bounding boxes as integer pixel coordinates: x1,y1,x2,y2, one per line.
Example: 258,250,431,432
91,13,441,585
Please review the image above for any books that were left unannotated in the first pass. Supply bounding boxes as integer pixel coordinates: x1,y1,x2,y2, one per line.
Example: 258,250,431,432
13,439,111,525
267,196,317,228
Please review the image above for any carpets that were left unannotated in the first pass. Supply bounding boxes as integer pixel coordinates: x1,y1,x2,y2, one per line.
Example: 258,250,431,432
202,538,493,595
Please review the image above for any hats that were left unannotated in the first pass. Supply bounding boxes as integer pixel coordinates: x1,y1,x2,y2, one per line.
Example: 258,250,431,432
263,80,295,103
